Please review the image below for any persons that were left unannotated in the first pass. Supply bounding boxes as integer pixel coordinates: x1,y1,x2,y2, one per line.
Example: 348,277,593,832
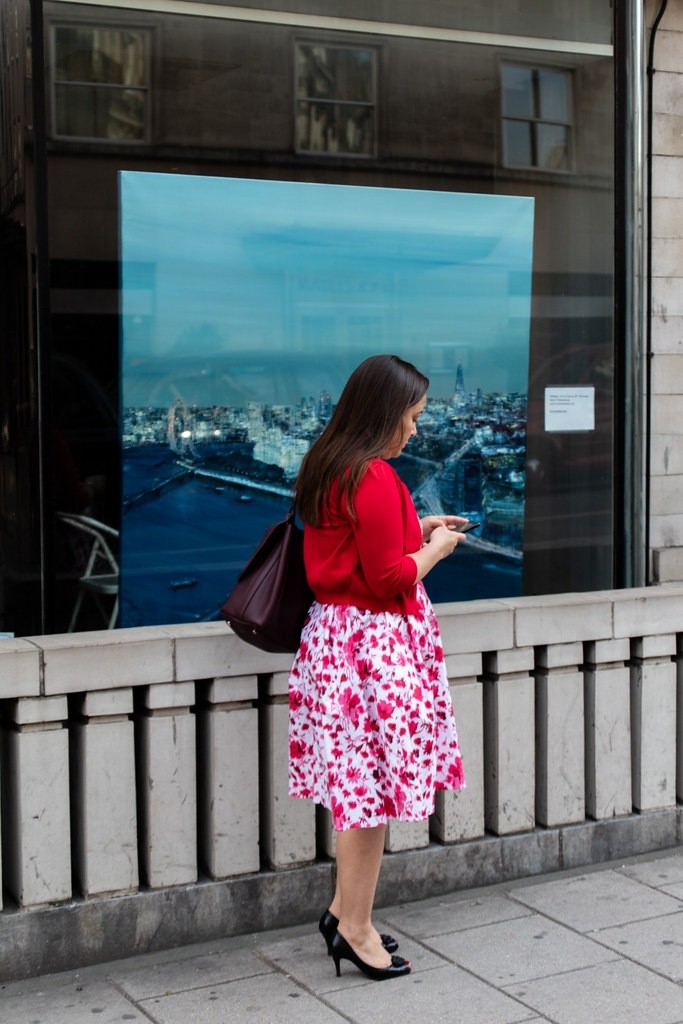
287,355,469,981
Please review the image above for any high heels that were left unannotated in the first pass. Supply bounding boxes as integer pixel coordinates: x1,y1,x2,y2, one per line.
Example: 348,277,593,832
318,909,399,957
330,927,413,982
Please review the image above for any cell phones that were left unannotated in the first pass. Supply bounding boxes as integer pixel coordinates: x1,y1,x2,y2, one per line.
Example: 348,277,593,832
425,521,481,544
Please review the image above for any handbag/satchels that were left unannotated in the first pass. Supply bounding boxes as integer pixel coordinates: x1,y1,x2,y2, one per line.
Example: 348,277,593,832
223,503,315,653
